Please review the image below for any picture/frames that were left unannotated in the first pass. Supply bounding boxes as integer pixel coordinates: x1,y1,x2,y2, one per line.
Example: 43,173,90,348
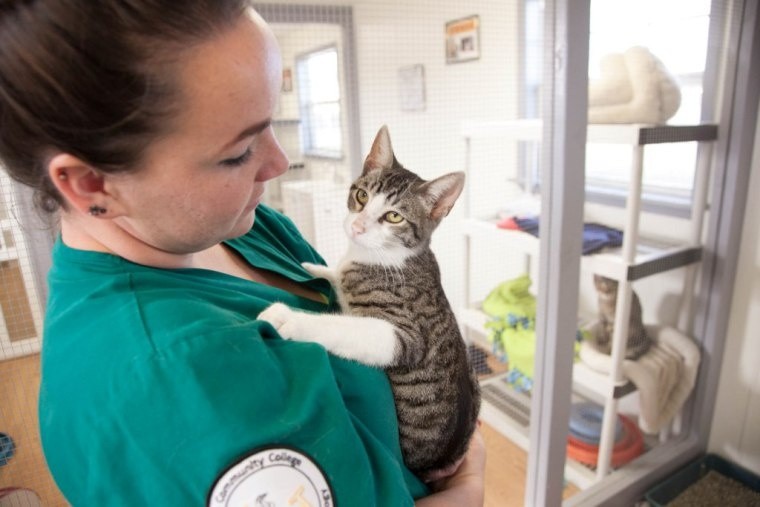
443,12,485,66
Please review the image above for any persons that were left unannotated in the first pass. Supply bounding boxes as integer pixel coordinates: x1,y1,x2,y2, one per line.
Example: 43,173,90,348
0,0,489,507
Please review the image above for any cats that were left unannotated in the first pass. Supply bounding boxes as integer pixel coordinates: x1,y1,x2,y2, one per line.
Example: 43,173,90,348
255,125,481,494
593,273,654,359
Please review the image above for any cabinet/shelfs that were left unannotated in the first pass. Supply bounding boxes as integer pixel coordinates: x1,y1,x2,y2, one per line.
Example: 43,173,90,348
437,97,725,496
271,115,306,171
281,178,346,272
